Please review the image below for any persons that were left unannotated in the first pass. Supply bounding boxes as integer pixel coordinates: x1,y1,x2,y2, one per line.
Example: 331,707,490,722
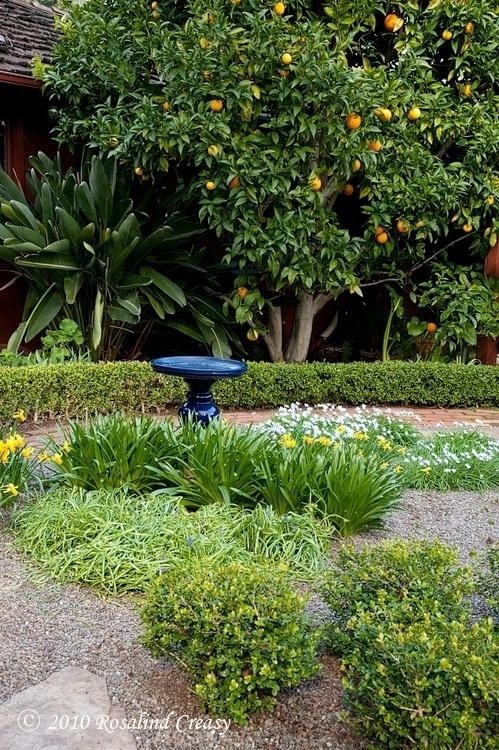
310,261,437,361
